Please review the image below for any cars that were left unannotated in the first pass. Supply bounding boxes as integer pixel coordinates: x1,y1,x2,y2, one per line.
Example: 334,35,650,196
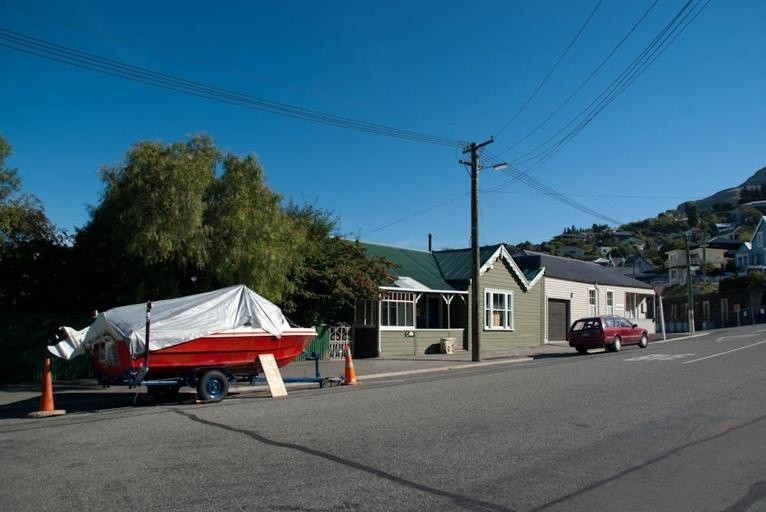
568,314,649,353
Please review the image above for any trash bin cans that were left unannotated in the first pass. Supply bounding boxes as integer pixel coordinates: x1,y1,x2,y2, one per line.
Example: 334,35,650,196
440,337,457,354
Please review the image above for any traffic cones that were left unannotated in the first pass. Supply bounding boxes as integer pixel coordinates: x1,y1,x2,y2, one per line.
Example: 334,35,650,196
27,359,67,419
339,343,363,386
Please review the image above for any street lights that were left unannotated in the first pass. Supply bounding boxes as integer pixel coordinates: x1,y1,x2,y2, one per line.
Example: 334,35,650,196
478,163,508,171
